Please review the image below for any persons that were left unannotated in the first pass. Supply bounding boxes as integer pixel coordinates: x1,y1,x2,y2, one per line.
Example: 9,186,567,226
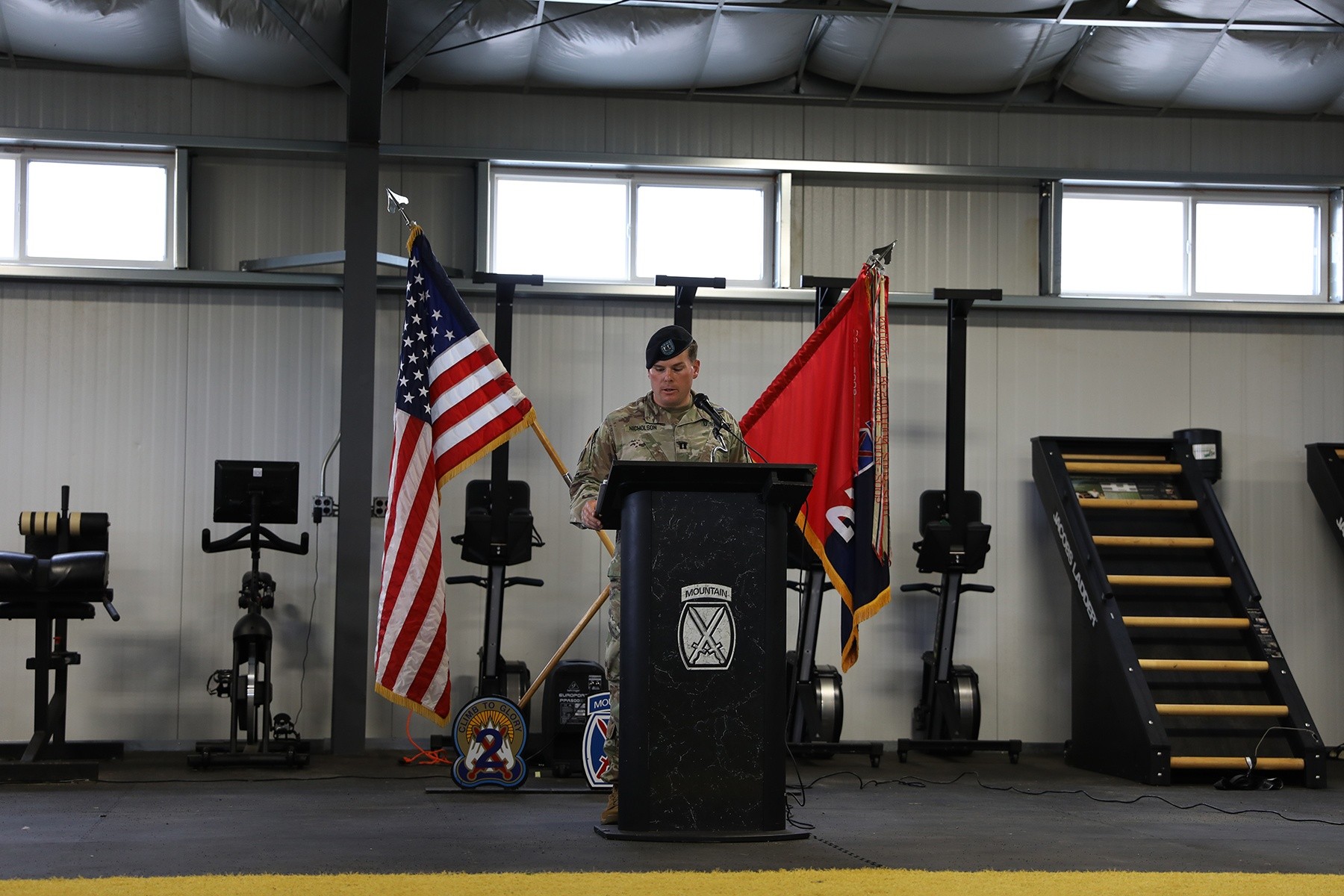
567,325,743,822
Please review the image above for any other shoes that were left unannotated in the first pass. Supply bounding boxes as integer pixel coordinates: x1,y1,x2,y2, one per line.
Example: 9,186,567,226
600,785,619,824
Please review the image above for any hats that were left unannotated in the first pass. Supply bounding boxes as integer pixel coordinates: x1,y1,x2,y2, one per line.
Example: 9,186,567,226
645,326,693,368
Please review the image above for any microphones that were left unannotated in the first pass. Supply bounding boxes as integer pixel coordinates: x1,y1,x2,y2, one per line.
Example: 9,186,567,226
693,393,731,432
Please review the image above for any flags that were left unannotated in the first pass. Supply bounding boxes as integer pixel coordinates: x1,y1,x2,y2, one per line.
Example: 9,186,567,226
740,263,893,674
374,231,535,724
